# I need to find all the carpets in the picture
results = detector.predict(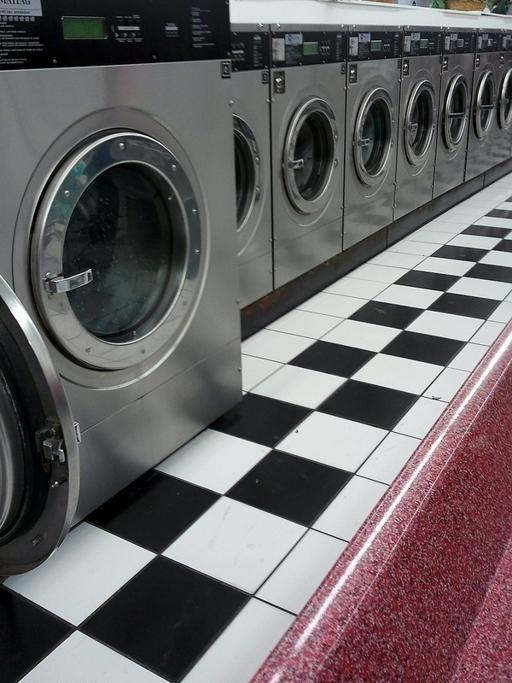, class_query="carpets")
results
[249,320,512,683]
